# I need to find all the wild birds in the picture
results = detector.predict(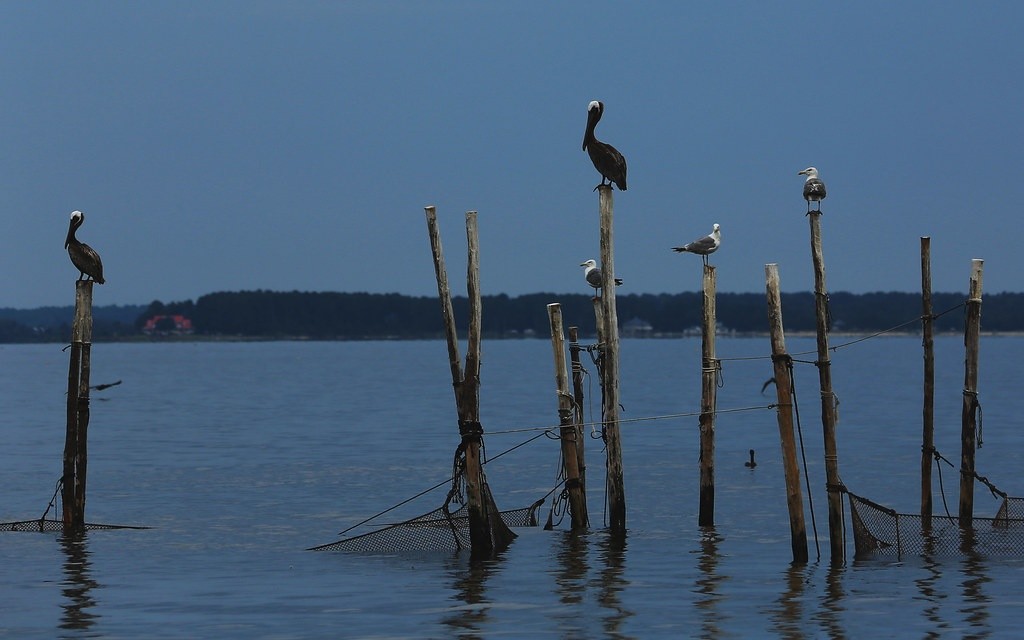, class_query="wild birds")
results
[582,101,627,192]
[581,260,623,299]
[671,223,720,266]
[797,167,826,217]
[65,210,106,284]
